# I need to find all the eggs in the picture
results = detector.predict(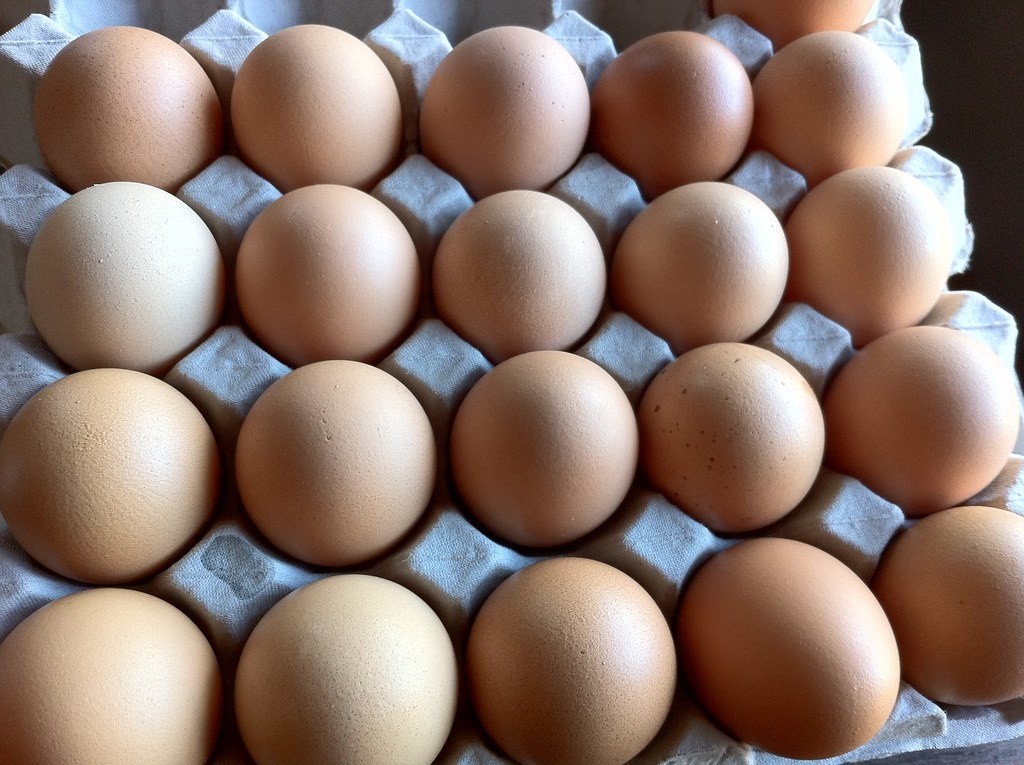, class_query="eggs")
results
[0,0,1024,764]
